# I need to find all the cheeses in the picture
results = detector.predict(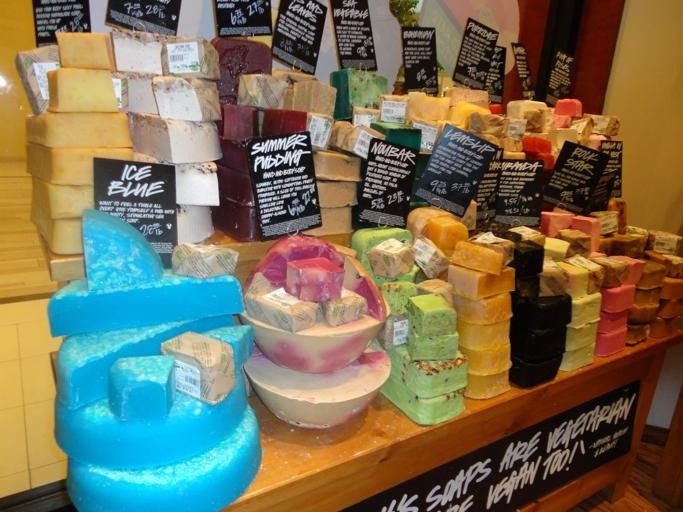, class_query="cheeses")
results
[14,28,683,512]
